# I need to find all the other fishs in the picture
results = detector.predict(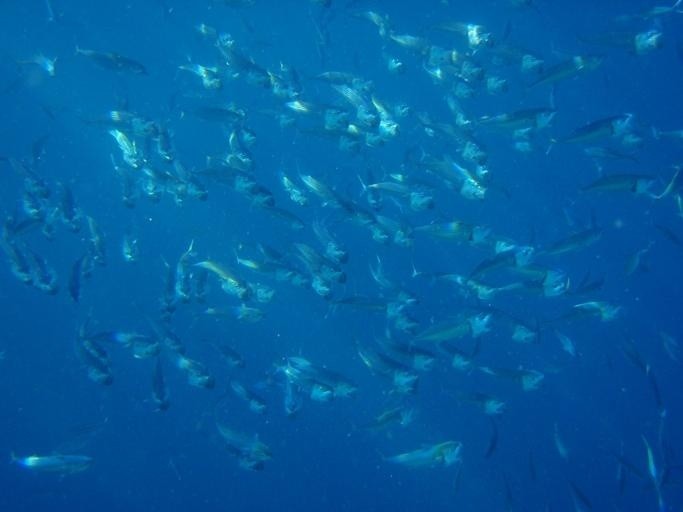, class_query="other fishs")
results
[0,0,683,512]
